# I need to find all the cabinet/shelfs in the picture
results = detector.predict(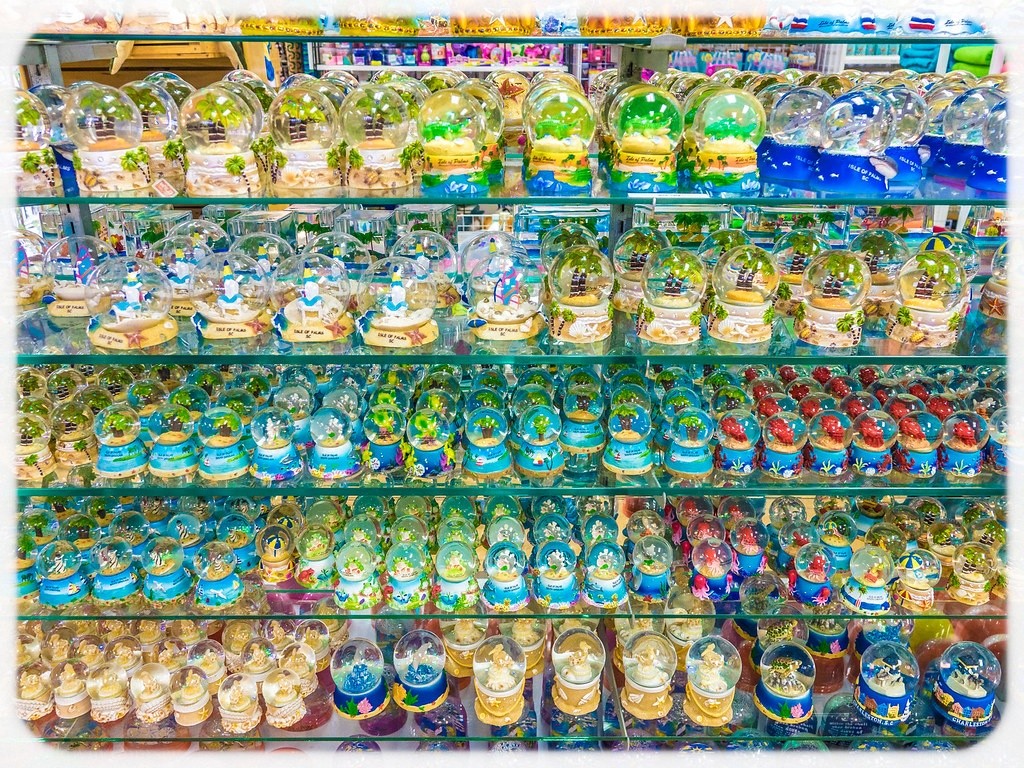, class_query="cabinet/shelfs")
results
[2,1,1022,754]
